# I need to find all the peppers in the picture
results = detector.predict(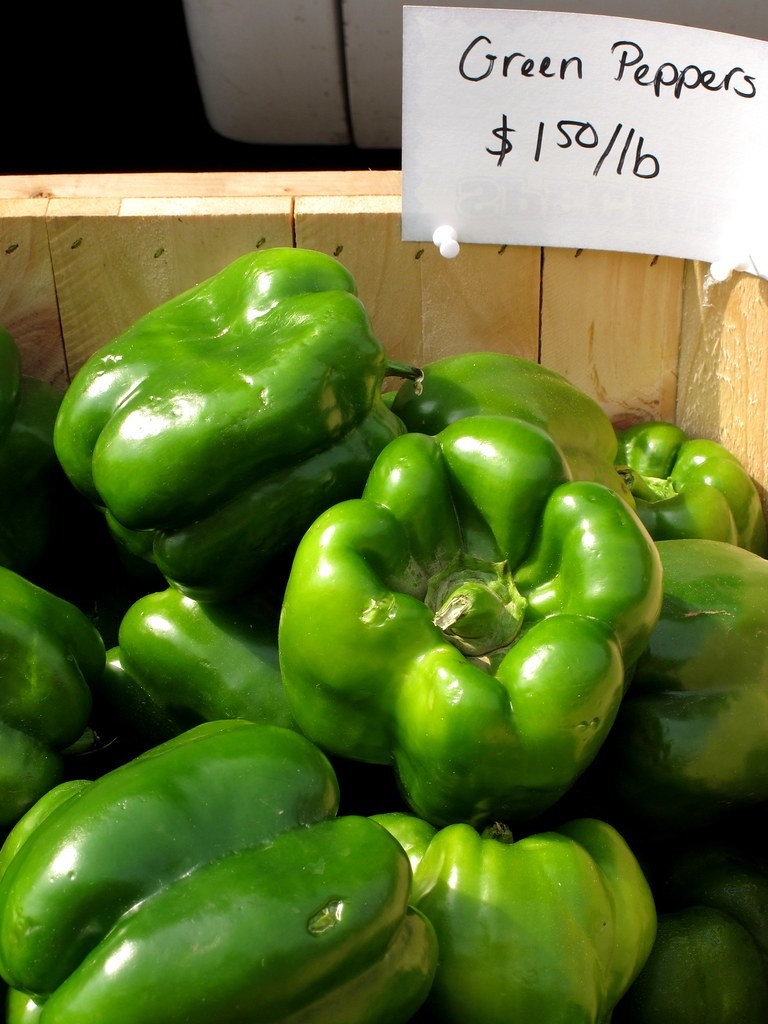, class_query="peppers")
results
[0,248,768,1024]
